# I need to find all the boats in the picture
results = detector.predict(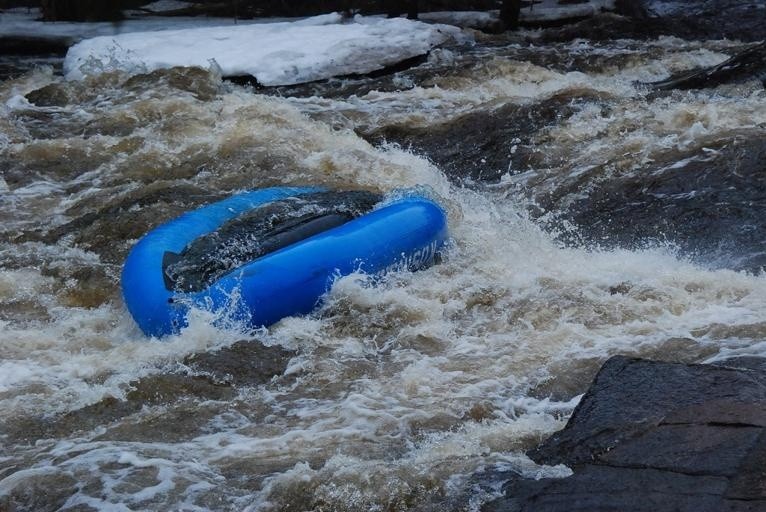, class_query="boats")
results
[118,177,449,347]
